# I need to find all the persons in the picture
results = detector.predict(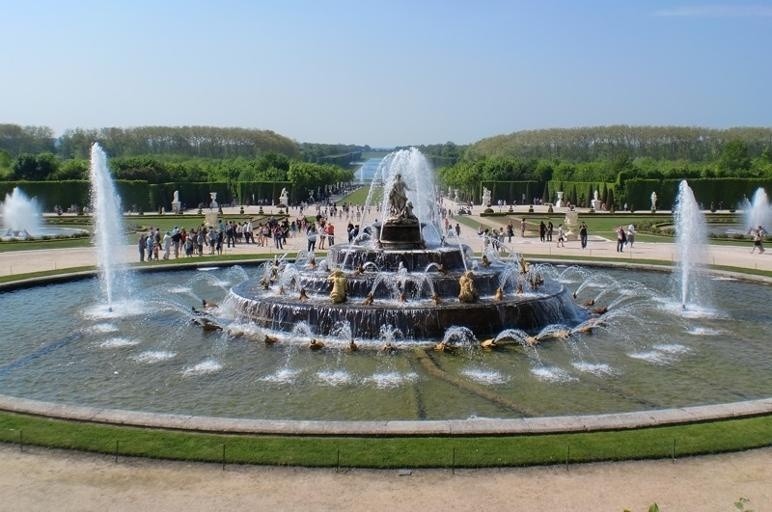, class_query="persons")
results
[578,221,588,248]
[460,271,479,299]
[616,223,636,252]
[498,193,525,207]
[750,225,765,254]
[290,184,383,252]
[533,196,572,211]
[139,216,289,261]
[391,176,415,218]
[539,221,565,247]
[436,195,473,236]
[328,270,348,299]
[478,218,526,252]
